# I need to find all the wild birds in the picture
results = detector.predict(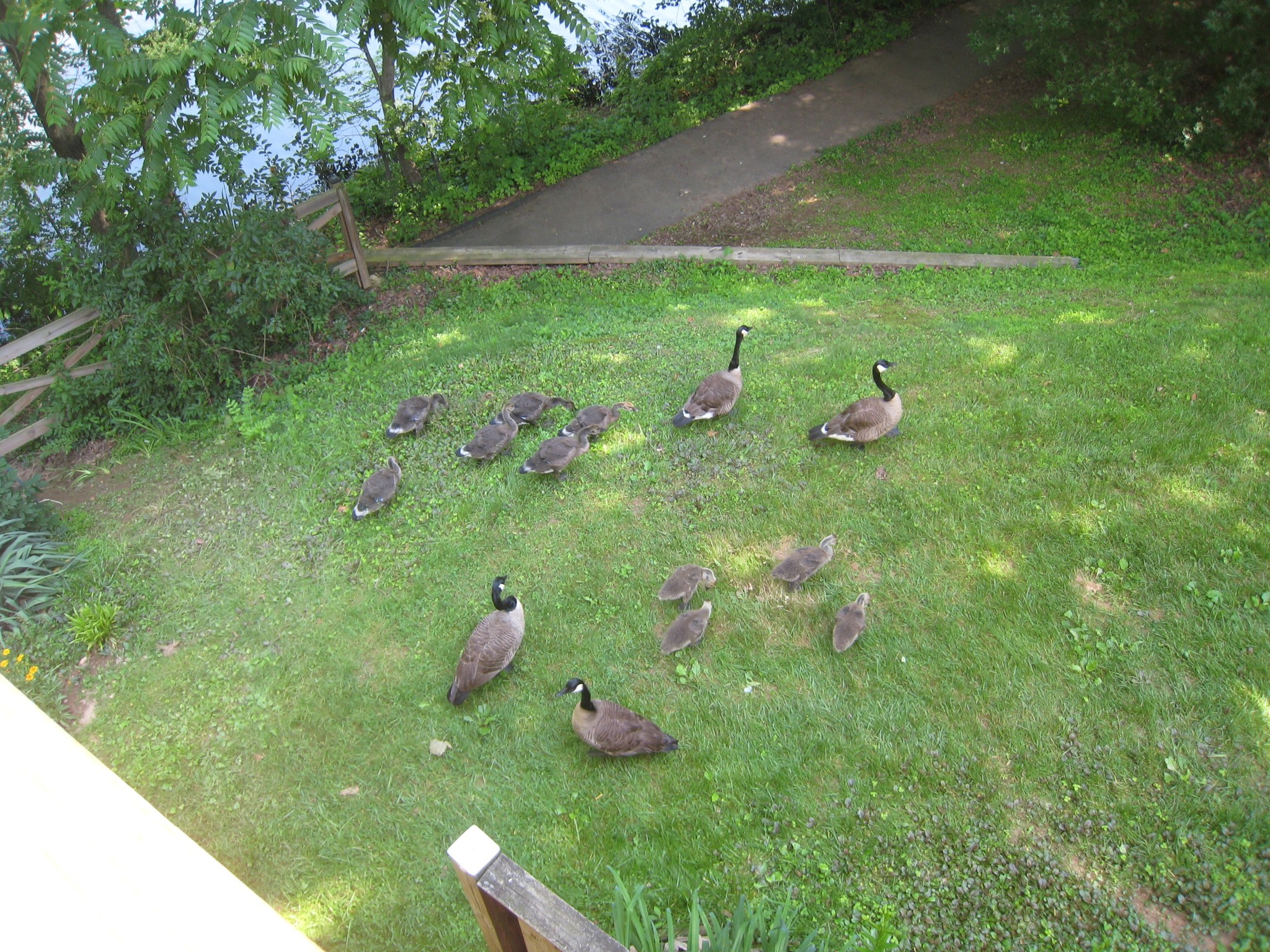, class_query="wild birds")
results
[555,678,679,757]
[808,360,903,450]
[455,402,519,469]
[772,534,838,592]
[833,592,870,652]
[386,393,449,439]
[558,401,639,437]
[657,564,716,611]
[672,325,754,429]
[519,424,608,481]
[351,456,402,521]
[447,574,526,706]
[489,392,577,428]
[660,599,712,653]
